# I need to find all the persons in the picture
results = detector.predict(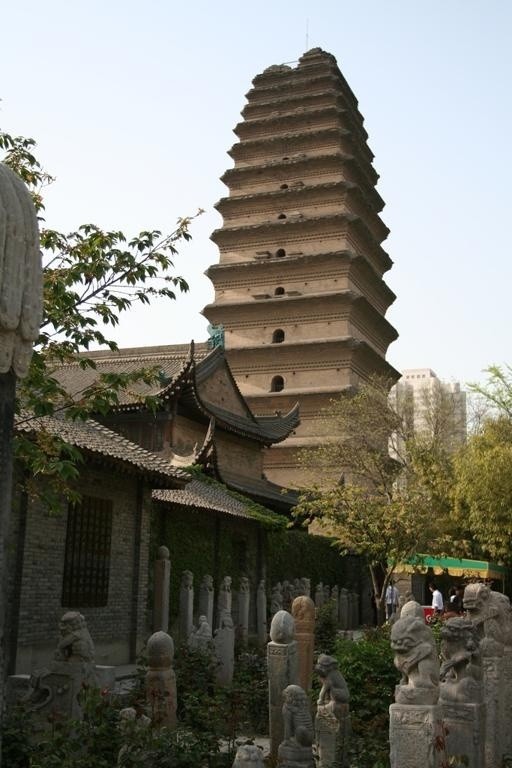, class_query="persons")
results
[428,582,443,626]
[385,579,398,619]
[447,586,462,616]
